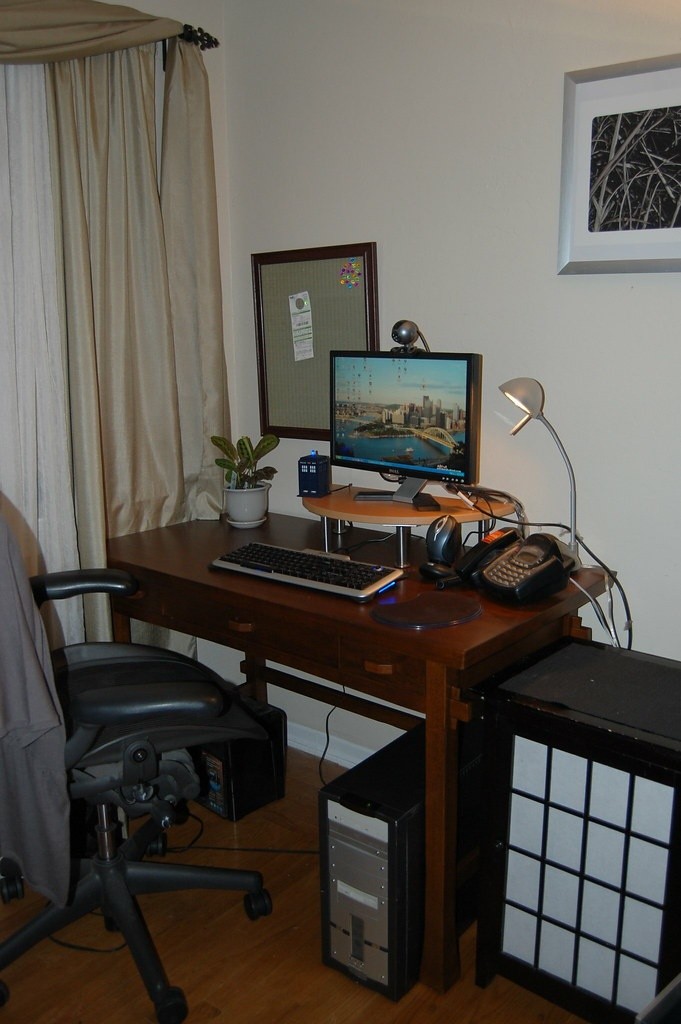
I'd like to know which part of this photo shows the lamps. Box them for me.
[497,377,582,572]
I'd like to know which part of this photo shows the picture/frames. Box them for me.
[250,242,380,442]
[556,54,681,276]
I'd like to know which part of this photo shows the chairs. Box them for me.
[0,518,274,1024]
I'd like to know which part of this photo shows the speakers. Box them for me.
[425,515,462,568]
[197,697,287,823]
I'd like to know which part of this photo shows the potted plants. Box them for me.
[211,433,280,529]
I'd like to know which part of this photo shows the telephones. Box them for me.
[454,526,575,604]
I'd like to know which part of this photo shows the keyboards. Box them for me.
[208,541,404,602]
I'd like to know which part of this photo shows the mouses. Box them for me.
[419,562,457,581]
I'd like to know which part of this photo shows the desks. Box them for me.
[105,513,617,997]
[301,485,515,569]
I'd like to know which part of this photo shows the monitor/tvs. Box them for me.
[330,350,483,511]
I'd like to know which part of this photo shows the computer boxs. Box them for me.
[318,722,488,1003]
